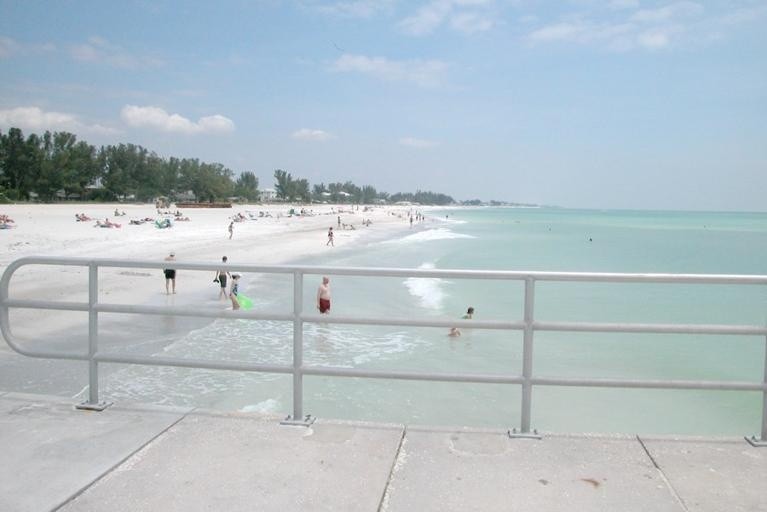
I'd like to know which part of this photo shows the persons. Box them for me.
[316,276,331,315]
[0,214,14,228]
[450,328,461,336]
[461,306,474,320]
[229,272,243,310]
[163,252,175,294]
[214,256,232,301]
